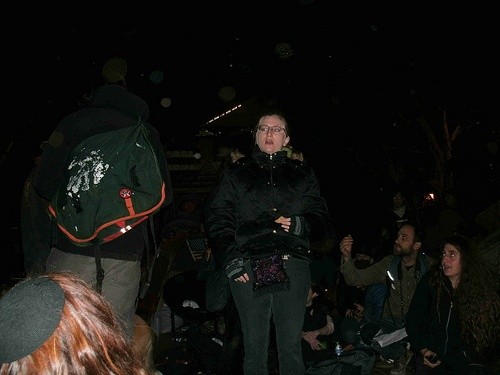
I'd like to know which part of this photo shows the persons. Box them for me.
[405,233,500,375]
[162,190,420,361]
[0,271,164,375]
[30,55,174,315]
[207,112,331,375]
[338,223,438,375]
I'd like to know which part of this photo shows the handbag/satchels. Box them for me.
[46,112,173,247]
[360,319,408,359]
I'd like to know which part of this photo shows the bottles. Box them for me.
[334,341,342,360]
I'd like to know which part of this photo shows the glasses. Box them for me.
[259,125,285,133]
